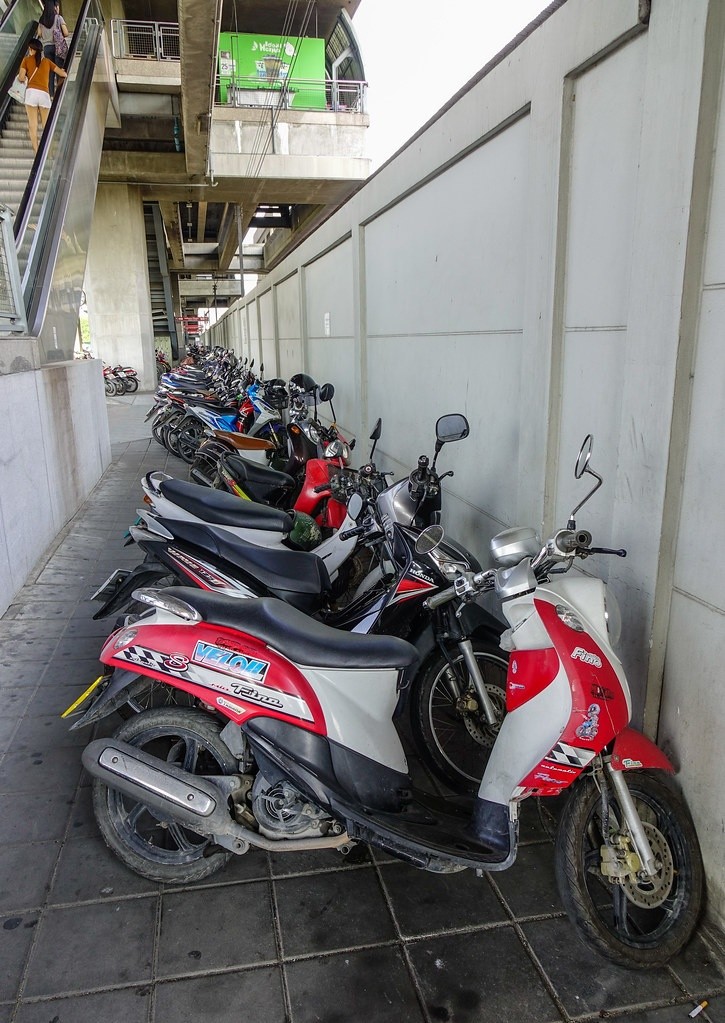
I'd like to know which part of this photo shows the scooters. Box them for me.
[62,430,706,974]
[89,342,512,800]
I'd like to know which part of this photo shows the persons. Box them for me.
[38,0,69,100]
[19,39,68,152]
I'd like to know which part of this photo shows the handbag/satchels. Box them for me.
[7,73,28,103]
[52,29,68,59]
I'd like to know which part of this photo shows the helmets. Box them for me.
[264,378,288,399]
[285,508,322,552]
[289,373,317,396]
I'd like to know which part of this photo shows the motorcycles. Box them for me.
[76,352,140,398]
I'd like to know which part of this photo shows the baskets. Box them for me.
[264,395,288,410]
